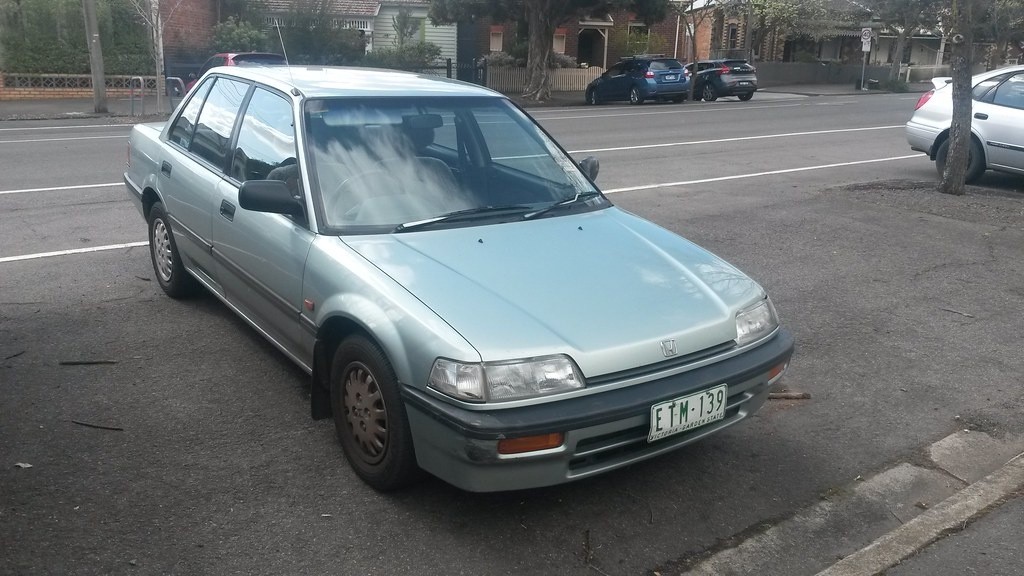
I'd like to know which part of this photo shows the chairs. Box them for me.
[373,157,460,195]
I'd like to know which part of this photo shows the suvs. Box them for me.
[585,56,692,106]
[185,51,289,93]
[683,58,759,102]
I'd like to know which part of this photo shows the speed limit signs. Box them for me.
[861,27,873,41]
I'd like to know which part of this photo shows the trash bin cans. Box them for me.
[817,57,841,84]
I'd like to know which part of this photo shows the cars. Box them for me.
[903,64,1024,184]
[121,64,798,494]
